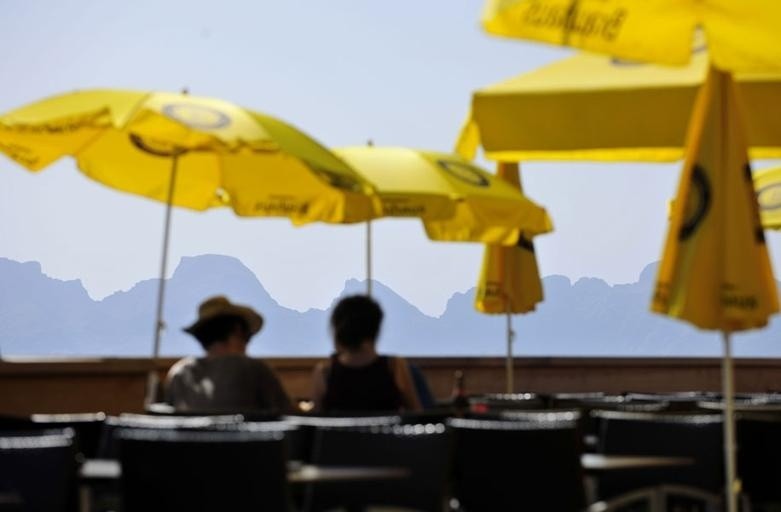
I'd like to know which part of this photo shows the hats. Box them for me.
[184,296,262,335]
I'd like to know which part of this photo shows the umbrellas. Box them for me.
[475,155,547,395]
[450,51,781,172]
[0,84,392,406]
[650,62,781,512]
[472,0,781,69]
[328,134,556,296]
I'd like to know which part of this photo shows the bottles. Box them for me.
[450,370,470,419]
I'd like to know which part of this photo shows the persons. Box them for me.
[309,292,425,415]
[164,294,294,422]
